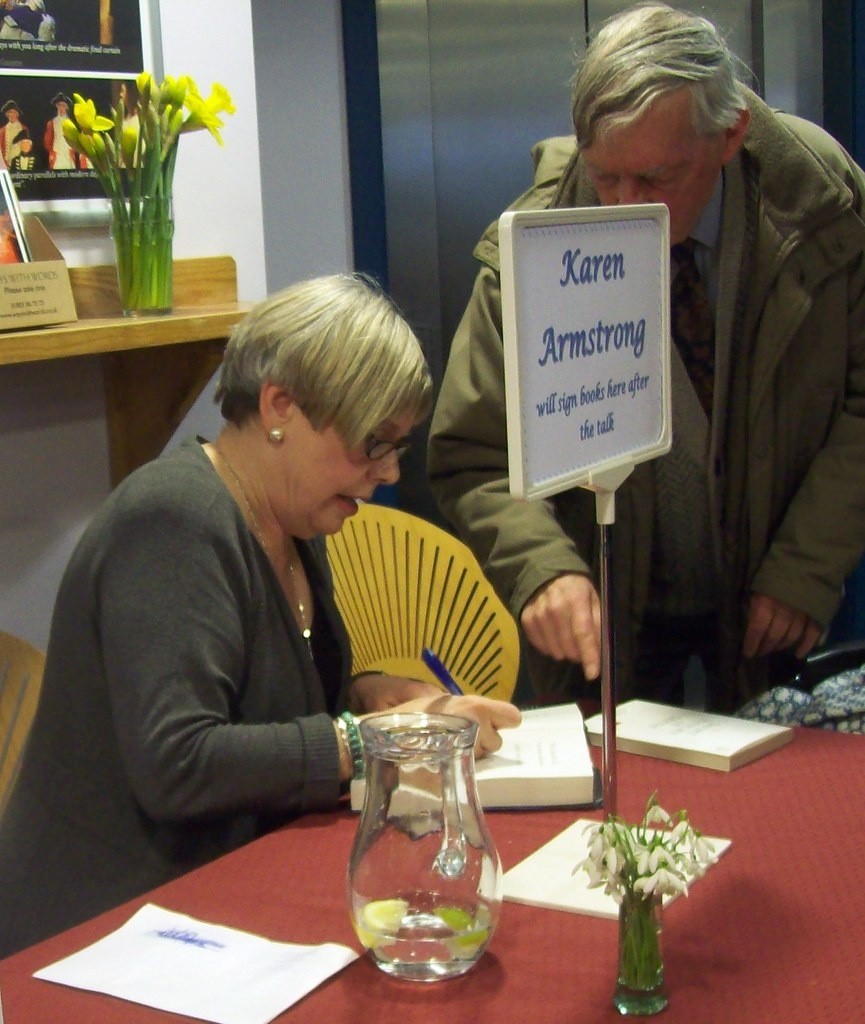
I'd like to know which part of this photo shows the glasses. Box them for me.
[362,432,413,462]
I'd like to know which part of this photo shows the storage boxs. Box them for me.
[0,216,80,331]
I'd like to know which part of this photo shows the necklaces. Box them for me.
[233,471,313,663]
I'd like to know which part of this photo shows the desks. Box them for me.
[0,699,865,1024]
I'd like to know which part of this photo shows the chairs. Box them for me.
[0,631,51,812]
[326,504,523,704]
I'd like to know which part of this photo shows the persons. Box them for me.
[1,274,521,960]
[422,5,865,717]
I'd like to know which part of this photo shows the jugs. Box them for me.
[345,712,504,982]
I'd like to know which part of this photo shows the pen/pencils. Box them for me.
[421,646,465,697]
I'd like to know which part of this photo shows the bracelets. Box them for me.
[336,712,364,777]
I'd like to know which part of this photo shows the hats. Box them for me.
[1,101,24,121]
[51,93,72,107]
[13,130,36,144]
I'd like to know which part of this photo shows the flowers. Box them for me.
[60,74,237,312]
[577,792,720,986]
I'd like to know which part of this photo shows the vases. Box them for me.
[104,194,175,318]
[613,893,670,1015]
[343,709,506,985]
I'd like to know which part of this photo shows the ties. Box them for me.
[670,237,716,426]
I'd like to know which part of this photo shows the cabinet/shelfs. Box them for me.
[0,256,256,487]
